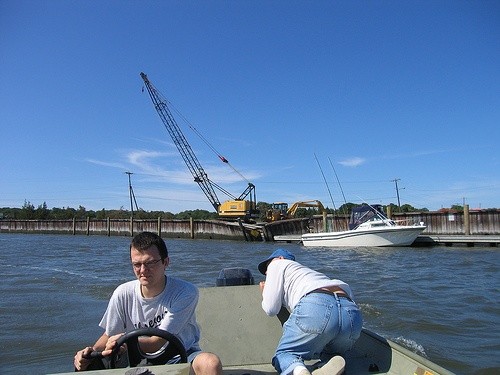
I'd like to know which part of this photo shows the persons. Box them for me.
[257,249,362,375]
[75,232,222,375]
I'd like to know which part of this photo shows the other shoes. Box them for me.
[293,365,312,375]
[311,355,345,375]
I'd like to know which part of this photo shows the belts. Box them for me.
[306,289,353,302]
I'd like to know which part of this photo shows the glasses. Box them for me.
[133,259,163,270]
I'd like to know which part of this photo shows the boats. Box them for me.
[301,152,427,247]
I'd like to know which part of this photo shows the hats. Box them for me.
[257,248,295,276]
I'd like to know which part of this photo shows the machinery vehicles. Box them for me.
[263,200,325,221]
[140,71,261,222]
